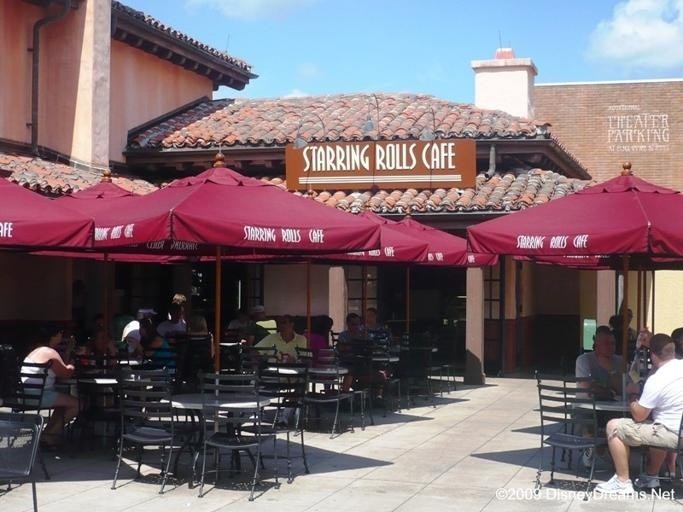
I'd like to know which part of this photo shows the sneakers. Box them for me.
[374,398,385,407]
[279,407,293,426]
[633,473,661,488]
[595,473,635,494]
[581,448,593,468]
[663,472,675,483]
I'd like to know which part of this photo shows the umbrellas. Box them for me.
[515,256,682,332]
[465,160,682,369]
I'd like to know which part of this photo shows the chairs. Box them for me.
[310,325,456,440]
[531,368,610,501]
[561,376,597,472]
[635,348,653,371]
[636,411,683,497]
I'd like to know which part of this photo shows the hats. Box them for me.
[252,305,265,313]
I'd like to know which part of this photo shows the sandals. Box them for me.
[39,428,66,451]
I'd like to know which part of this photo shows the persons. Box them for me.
[572,309,682,468]
[18,302,191,459]
[593,333,682,494]
[229,306,430,409]
